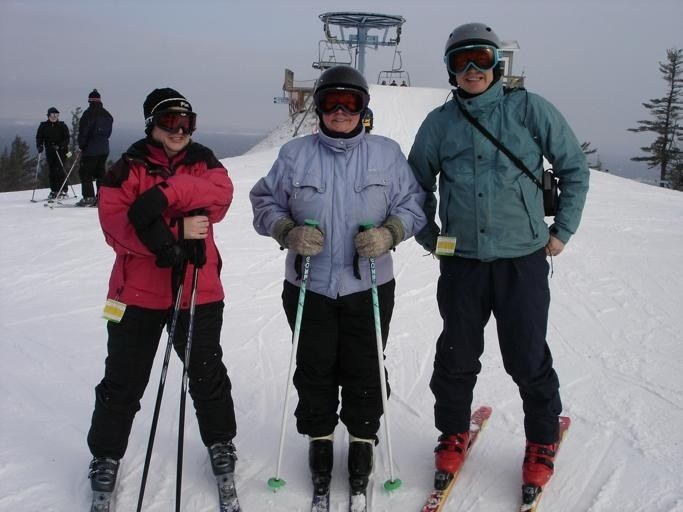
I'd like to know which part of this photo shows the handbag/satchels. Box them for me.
[542,169,560,216]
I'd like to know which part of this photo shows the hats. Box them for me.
[88,88,101,102]
[143,87,192,125]
[47,107,59,116]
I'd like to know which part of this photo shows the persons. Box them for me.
[85,85,238,494]
[380,79,386,85]
[405,21,592,504]
[248,62,427,496]
[35,106,70,198]
[77,88,113,205]
[399,79,406,86]
[389,79,397,85]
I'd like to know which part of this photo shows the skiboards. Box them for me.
[310,474,368,512]
[90,473,240,512]
[30,196,97,208]
[420,406,569,512]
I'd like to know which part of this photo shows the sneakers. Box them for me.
[89,454,127,491]
[48,191,68,199]
[305,430,337,476]
[346,431,380,476]
[75,196,99,207]
[206,438,239,479]
[520,434,558,488]
[432,427,471,475]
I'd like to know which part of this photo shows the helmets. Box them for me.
[313,62,371,117]
[442,21,504,57]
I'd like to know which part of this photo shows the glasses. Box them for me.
[155,110,196,134]
[442,44,500,75]
[49,112,59,117]
[314,87,369,116]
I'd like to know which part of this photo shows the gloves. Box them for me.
[36,144,45,152]
[272,217,326,257]
[56,141,66,151]
[127,184,169,229]
[352,215,406,260]
